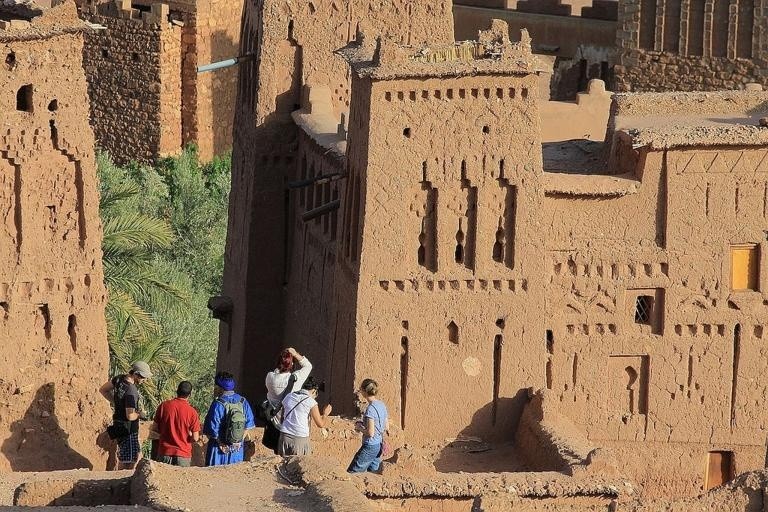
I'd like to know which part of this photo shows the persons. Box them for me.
[97,361,153,470]
[276,375,332,455]
[261,346,314,443]
[346,378,392,473]
[202,369,257,466]
[153,380,200,466]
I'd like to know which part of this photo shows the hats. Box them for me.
[133,361,153,378]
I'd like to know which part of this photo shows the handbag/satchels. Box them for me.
[107,420,130,440]
[260,400,275,423]
[379,441,390,458]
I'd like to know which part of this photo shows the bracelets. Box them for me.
[294,352,299,356]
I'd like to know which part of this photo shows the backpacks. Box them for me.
[213,396,246,446]
